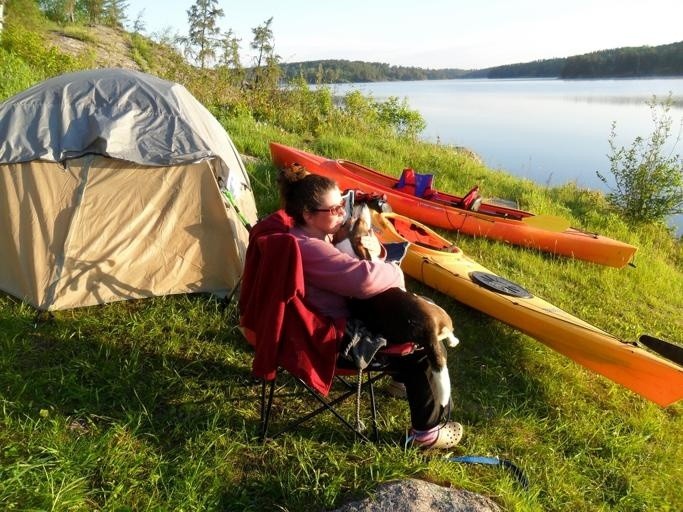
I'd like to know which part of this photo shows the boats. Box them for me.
[346,187,683,411]
[269,137,637,273]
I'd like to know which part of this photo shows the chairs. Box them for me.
[234,209,418,449]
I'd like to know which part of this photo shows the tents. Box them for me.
[0,67,259,313]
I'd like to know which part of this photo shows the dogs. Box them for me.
[330,203,459,425]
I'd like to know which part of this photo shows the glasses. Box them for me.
[313,200,346,215]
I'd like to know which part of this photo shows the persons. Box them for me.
[283,175,463,452]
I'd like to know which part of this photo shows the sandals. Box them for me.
[410,422,463,449]
